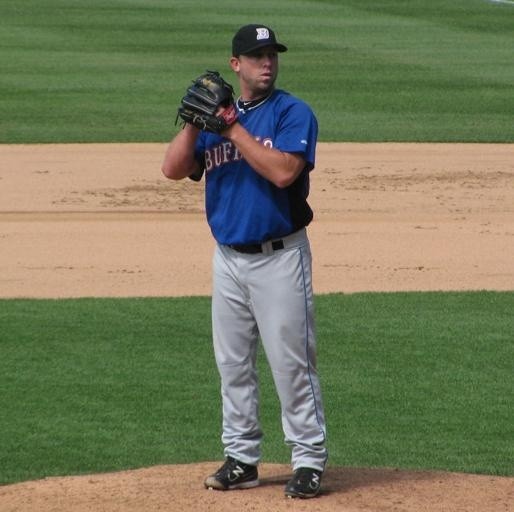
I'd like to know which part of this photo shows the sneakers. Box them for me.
[283,467,322,500]
[204,456,260,490]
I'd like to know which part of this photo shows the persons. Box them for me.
[159,23,330,501]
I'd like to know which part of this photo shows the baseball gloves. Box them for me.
[177,70,241,133]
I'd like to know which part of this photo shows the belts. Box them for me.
[215,239,283,254]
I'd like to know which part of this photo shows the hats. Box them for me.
[232,23,288,56]
